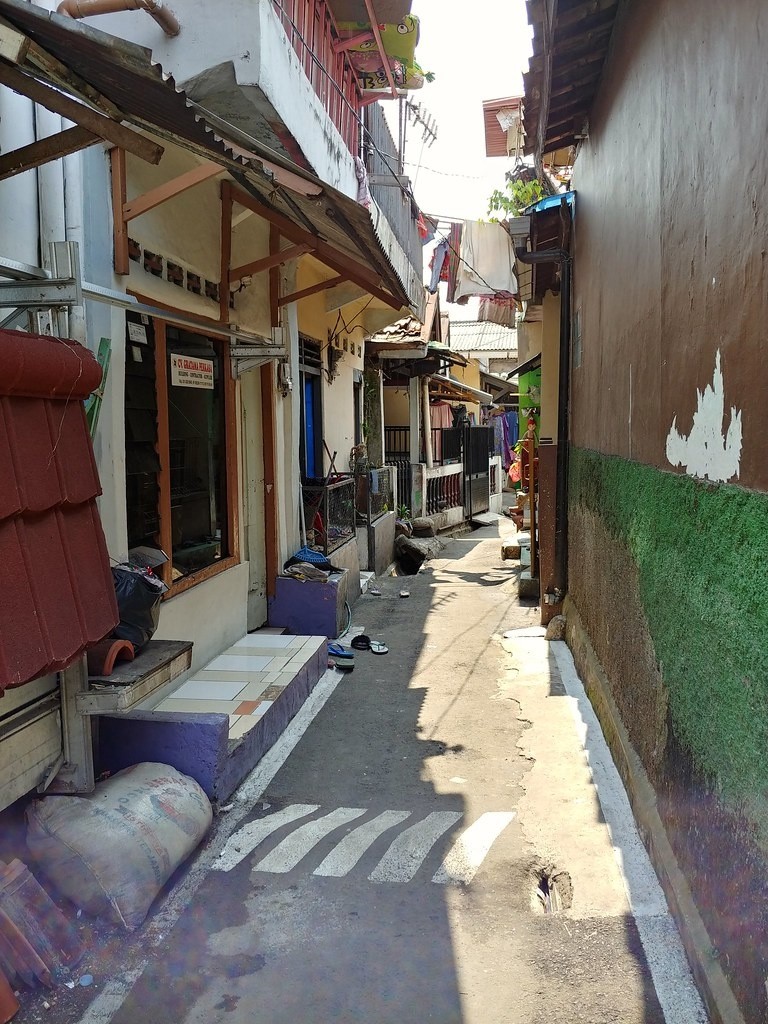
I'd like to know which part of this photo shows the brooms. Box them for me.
[282,481,346,575]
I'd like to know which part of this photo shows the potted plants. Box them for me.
[489,181,545,250]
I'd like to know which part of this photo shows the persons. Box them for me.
[522,420,538,444]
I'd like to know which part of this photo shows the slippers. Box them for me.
[328,636,389,669]
